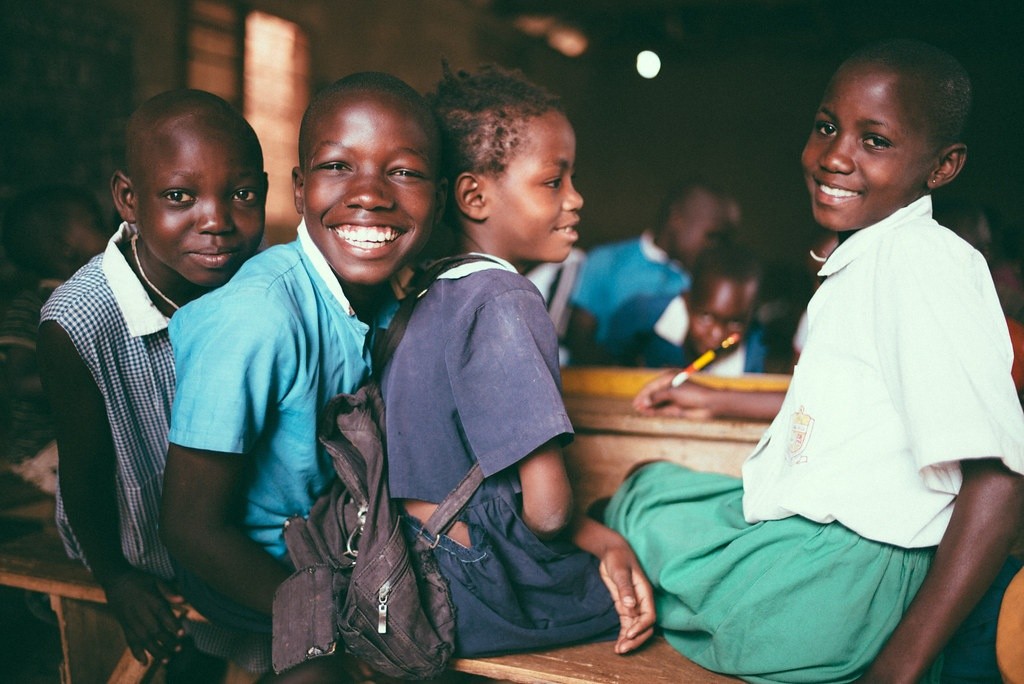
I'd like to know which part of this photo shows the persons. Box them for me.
[158,71,441,684]
[0,87,268,684]
[378,65,657,654]
[558,41,1024,684]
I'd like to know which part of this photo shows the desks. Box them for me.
[556,389,778,510]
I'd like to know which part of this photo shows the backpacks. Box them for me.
[270,253,508,682]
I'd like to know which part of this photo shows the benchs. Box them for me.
[0,526,750,684]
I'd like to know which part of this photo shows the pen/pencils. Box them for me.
[670,332,741,388]
